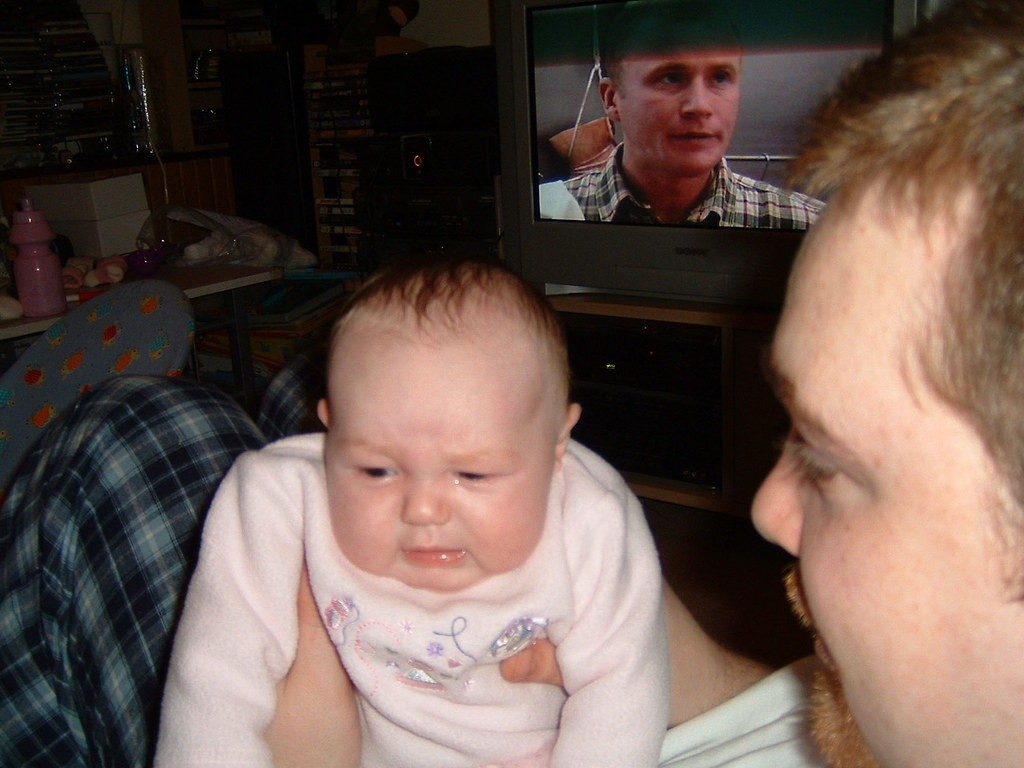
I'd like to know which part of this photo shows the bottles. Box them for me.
[10,196,67,317]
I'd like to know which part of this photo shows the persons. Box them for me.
[560,0,825,230]
[0,0,1024,768]
[153,251,669,768]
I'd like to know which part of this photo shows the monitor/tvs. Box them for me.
[492,0,919,309]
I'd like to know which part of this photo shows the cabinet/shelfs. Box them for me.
[546,291,791,519]
[140,0,232,150]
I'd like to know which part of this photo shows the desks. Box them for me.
[0,250,282,408]
